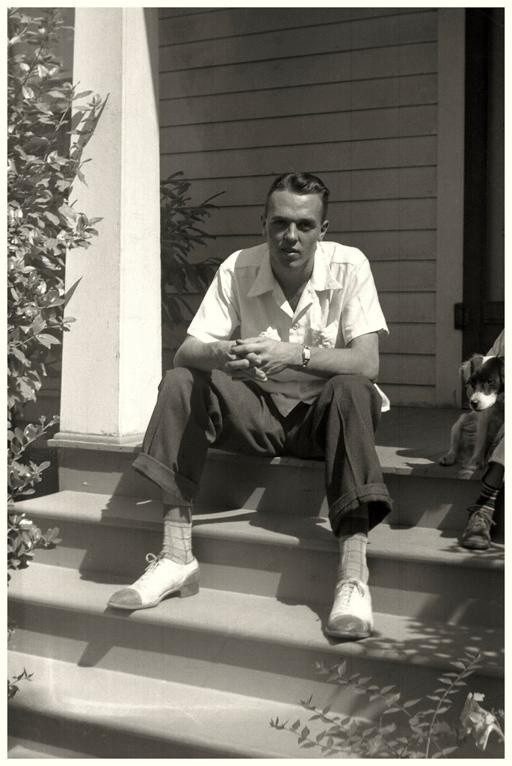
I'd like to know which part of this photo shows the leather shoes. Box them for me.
[327,577,373,638]
[107,554,201,611]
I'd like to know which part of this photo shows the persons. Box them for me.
[459,326,505,549]
[107,173,392,644]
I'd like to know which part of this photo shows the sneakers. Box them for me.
[460,504,495,549]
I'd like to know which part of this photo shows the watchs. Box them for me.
[297,344,312,369]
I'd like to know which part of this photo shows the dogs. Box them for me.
[436,353,504,471]
